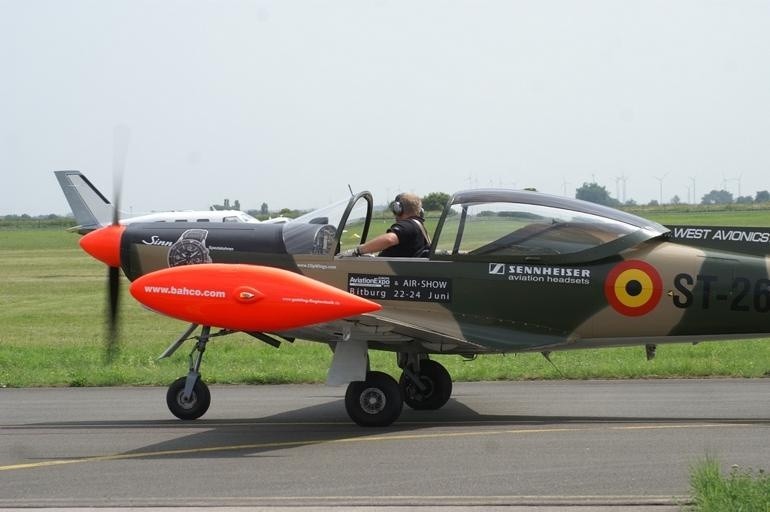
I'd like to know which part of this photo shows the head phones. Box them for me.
[389,193,424,218]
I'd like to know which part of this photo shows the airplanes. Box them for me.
[55,169,291,232]
[78,126,770,428]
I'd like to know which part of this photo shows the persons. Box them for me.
[337,191,432,256]
